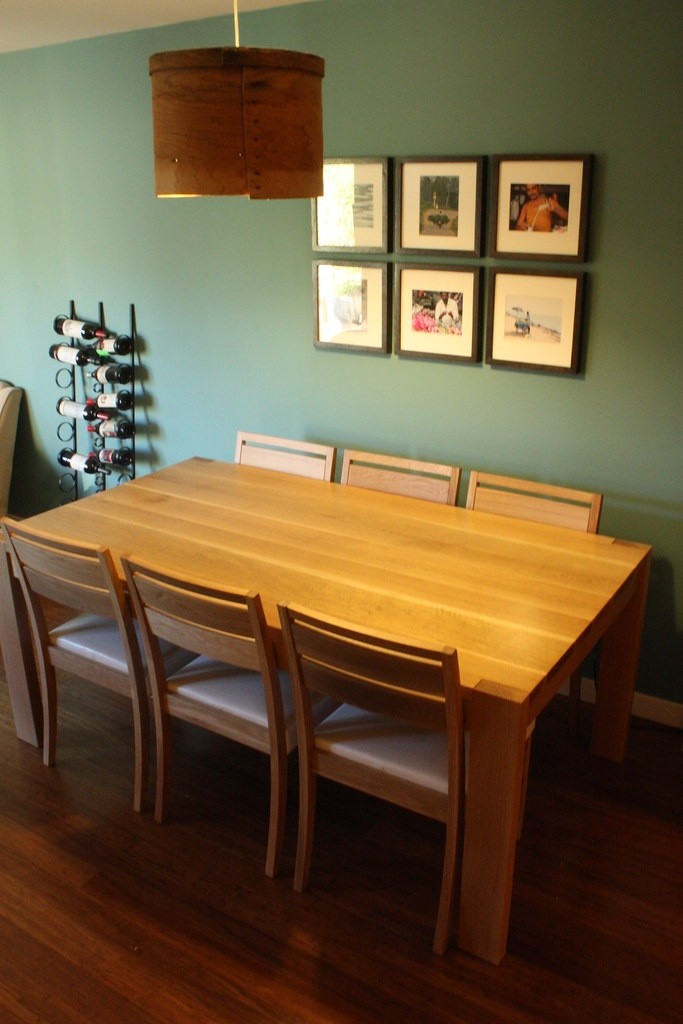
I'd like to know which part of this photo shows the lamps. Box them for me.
[149,0,325,201]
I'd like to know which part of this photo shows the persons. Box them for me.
[516,184,568,232]
[526,311,530,333]
[412,290,463,327]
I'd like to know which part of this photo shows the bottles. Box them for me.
[86,393,133,411]
[57,450,112,476]
[55,398,111,421]
[87,339,131,356]
[86,365,132,384]
[48,344,103,367]
[87,450,131,466]
[53,318,110,340]
[87,421,134,440]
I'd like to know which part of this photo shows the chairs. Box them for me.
[1,515,202,814]
[275,599,537,957]
[120,550,342,878]
[235,432,604,739]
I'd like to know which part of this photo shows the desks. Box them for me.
[0,455,652,966]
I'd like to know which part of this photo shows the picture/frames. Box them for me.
[486,266,585,373]
[489,153,592,264]
[394,155,489,258]
[393,261,486,363]
[310,156,393,255]
[311,257,392,356]
